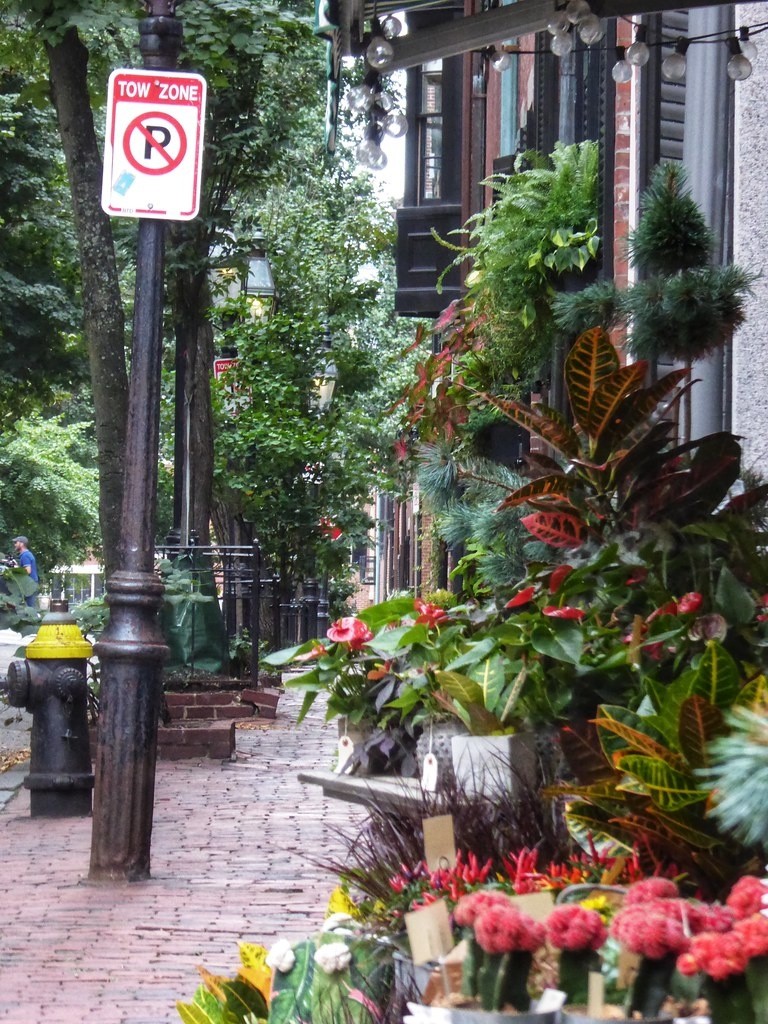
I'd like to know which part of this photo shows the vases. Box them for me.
[337,716,374,749]
[416,720,467,786]
[451,731,537,801]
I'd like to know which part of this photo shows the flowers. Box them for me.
[175,565,768,1024]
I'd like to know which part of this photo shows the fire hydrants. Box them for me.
[0,598,96,794]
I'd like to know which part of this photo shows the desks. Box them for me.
[297,771,434,820]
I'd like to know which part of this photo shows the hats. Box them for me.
[12,536,28,546]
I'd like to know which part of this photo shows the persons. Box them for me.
[12,536,38,608]
